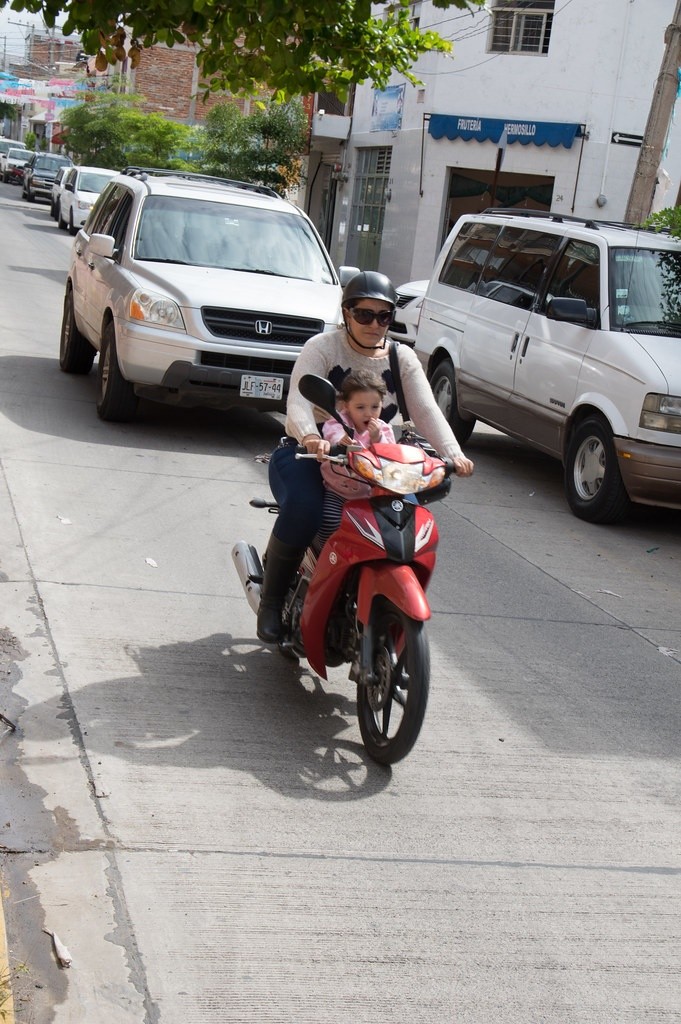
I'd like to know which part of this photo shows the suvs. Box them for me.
[412,207,681,524]
[58,165,343,422]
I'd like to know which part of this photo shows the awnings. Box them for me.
[427,112,584,149]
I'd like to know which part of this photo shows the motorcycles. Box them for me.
[231,373,473,765]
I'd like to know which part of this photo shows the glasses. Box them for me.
[348,307,396,328]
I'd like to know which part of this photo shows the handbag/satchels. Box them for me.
[395,419,452,506]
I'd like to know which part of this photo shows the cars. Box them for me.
[21,151,76,202]
[49,167,73,218]
[58,165,121,235]
[2,147,34,183]
[0,135,27,181]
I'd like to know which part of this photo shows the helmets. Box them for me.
[341,271,397,312]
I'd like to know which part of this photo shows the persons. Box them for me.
[318,370,396,539]
[256,272,474,644]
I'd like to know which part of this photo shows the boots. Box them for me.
[257,532,305,644]
